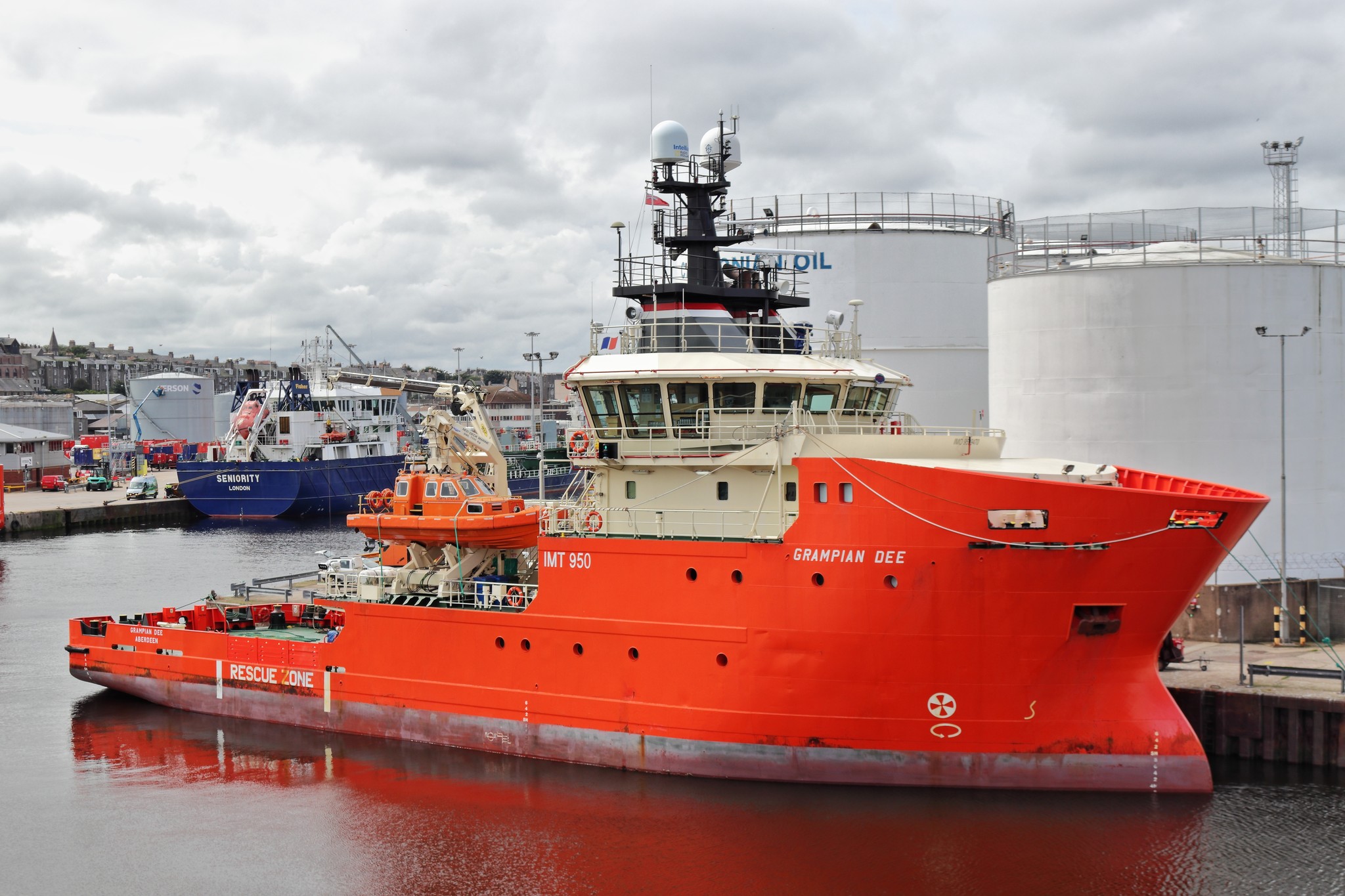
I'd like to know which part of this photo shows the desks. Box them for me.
[606,416,622,435]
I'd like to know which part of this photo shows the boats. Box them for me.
[319,430,347,441]
[346,452,569,550]
[64,63,1272,794]
[235,401,270,440]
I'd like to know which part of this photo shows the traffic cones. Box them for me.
[163,493,167,499]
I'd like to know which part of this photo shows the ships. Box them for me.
[176,325,588,518]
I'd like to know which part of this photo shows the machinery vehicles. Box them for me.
[86,462,114,491]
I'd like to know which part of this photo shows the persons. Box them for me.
[75,470,81,483]
[164,483,173,498]
[406,441,413,450]
[157,386,160,389]
[161,386,164,395]
[304,456,309,462]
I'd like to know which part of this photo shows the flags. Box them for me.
[645,193,669,206]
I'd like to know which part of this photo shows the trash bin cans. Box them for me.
[164,483,185,497]
[501,575,520,598]
[1172,639,1184,655]
[486,575,510,606]
[520,446,527,450]
[473,577,496,606]
[891,421,901,434]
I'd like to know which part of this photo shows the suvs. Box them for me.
[41,475,68,492]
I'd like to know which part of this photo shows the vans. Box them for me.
[126,476,158,501]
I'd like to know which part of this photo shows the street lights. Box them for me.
[101,458,104,468]
[524,331,540,438]
[453,348,465,385]
[1255,325,1312,643]
[522,352,559,500]
[347,344,357,377]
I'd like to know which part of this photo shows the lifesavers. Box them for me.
[570,430,588,452]
[586,512,602,532]
[304,457,308,461]
[381,488,394,509]
[366,490,383,509]
[507,586,523,606]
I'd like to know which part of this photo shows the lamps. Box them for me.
[632,469,771,475]
[1096,465,1107,474]
[1062,464,1074,474]
[720,195,727,210]
[763,208,774,220]
[826,310,844,333]
[602,444,609,459]
[1003,212,1013,218]
[652,169,659,182]
[658,188,664,193]
[625,306,644,324]
[355,527,359,533]
[693,177,698,183]
[599,493,603,496]
[1081,235,1088,241]
[725,140,732,154]
[593,322,603,332]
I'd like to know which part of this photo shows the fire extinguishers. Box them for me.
[655,279,657,284]
[633,421,639,427]
[654,170,657,182]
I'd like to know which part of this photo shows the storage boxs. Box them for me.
[63,435,221,465]
[280,440,288,444]
